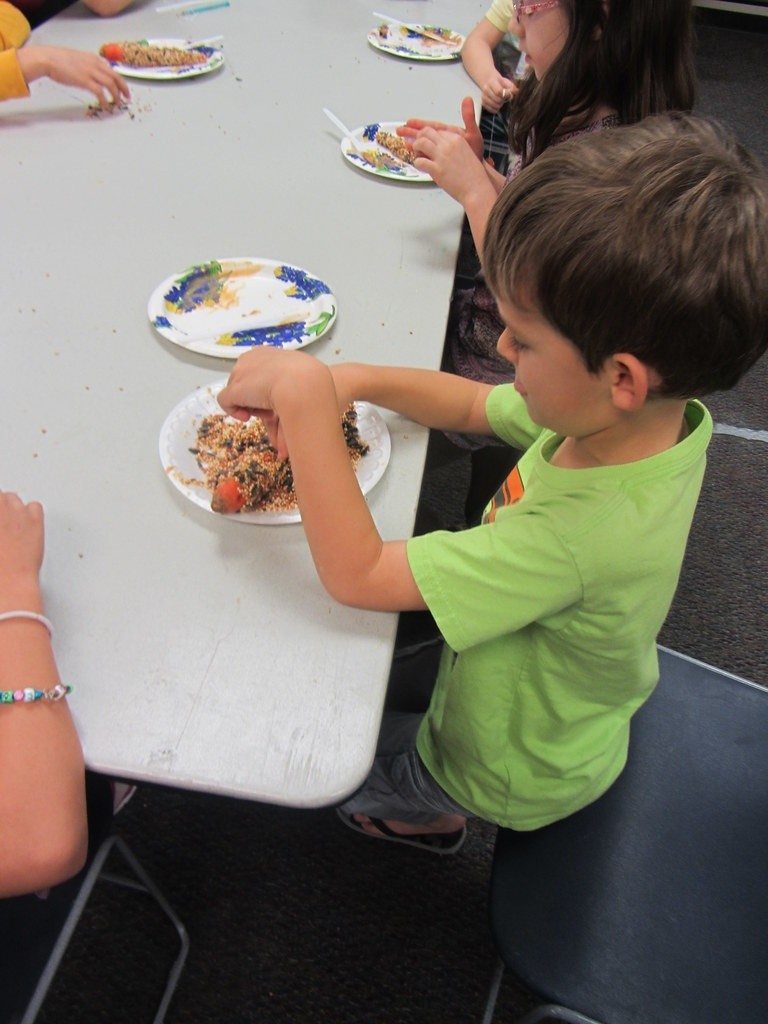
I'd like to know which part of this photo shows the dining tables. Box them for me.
[0,0,491,816]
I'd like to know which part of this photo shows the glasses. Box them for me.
[513,0,564,24]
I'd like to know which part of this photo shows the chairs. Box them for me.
[476,642,768,1024]
[1,831,194,1024]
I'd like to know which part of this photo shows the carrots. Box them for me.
[210,478,246,515]
[99,43,124,60]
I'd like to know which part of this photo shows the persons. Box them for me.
[396,0,693,529]
[0,490,137,898]
[218,109,768,853]
[0,0,130,112]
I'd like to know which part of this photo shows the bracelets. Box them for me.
[0,685,71,703]
[0,610,54,639]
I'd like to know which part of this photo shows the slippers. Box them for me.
[336,805,467,855]
